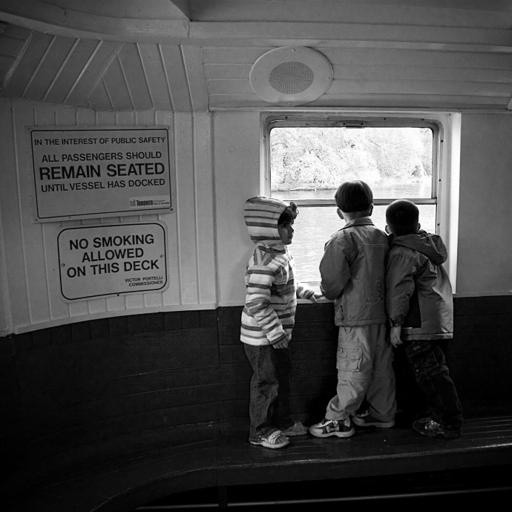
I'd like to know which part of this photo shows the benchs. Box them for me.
[0,294,512,511]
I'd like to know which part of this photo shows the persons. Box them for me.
[241,196,322,450]
[382,200,465,440]
[306,180,397,438]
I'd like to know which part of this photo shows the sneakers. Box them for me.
[309,406,460,439]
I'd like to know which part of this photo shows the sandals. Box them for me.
[249,420,308,449]
